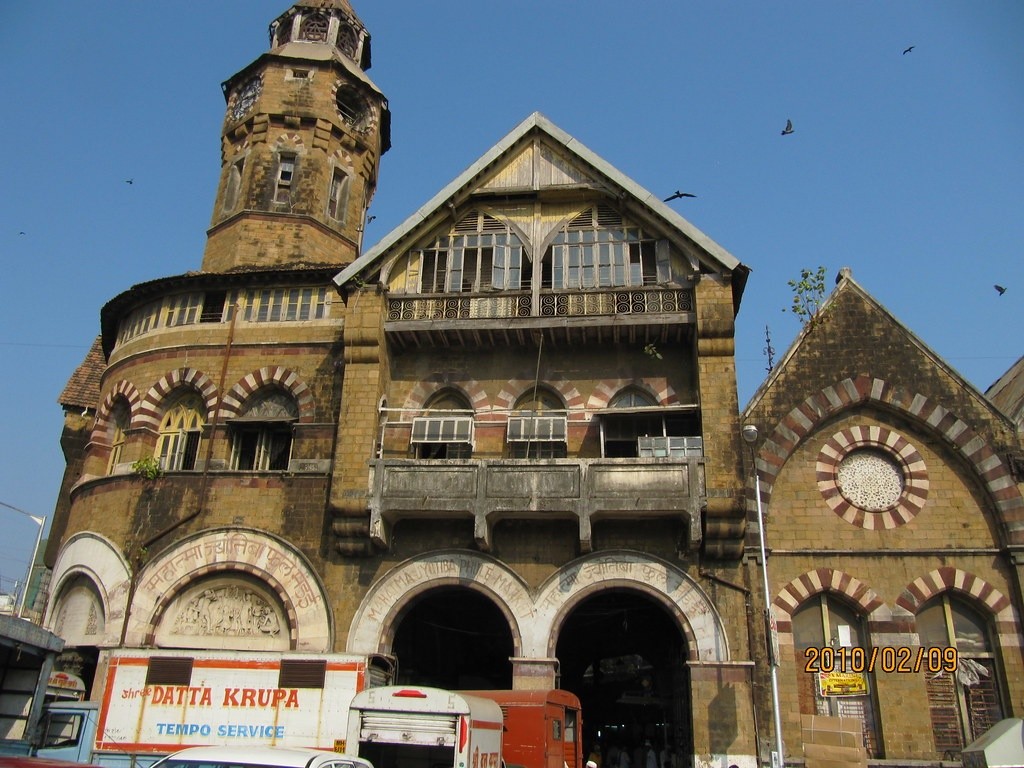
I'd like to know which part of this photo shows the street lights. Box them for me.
[741,424,787,768]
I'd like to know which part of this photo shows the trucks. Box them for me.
[344,685,508,768]
[31,646,398,768]
[449,688,582,768]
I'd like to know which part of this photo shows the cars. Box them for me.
[149,743,374,768]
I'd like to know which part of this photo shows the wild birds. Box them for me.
[126,178,134,185]
[19,231,25,236]
[902,45,915,56]
[781,118,795,137]
[366,214,377,224]
[664,190,696,203]
[992,283,1008,297]
